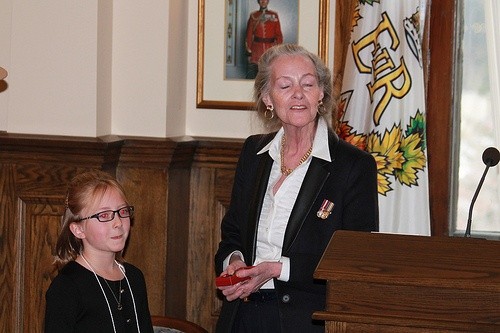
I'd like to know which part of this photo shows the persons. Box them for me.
[43,170,155,333]
[214,42,379,333]
[244,0,284,78]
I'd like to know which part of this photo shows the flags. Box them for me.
[331,1,429,238]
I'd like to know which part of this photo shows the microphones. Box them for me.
[464,147,500,237]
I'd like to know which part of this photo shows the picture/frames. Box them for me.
[196,0,326,110]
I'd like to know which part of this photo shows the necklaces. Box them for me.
[279,127,312,176]
[82,252,123,310]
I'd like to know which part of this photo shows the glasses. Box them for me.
[73,205,135,222]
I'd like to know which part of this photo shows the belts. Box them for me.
[241,291,278,303]
